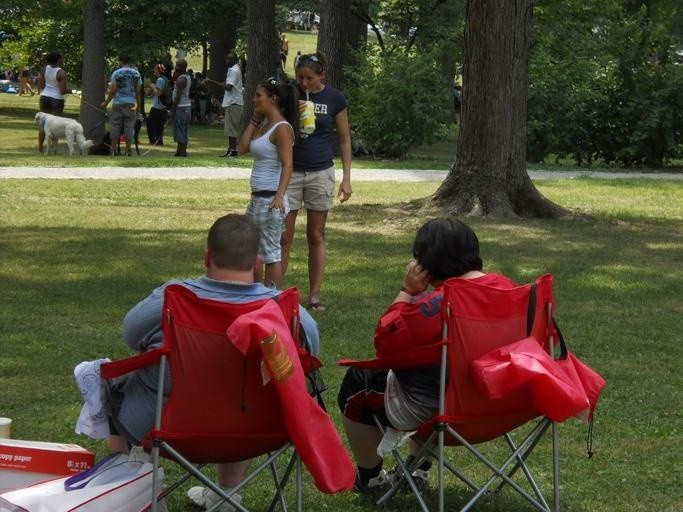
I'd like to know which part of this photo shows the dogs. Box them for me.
[34,112,95,156]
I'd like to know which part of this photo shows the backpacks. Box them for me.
[159,76,173,107]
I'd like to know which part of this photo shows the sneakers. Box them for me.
[392,463,430,492]
[186,485,243,512]
[345,468,391,494]
[220,150,238,158]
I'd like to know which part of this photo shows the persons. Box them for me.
[294,50,301,69]
[237,76,299,290]
[106,213,321,511]
[0,53,243,158]
[280,52,352,311]
[282,33,289,70]
[337,217,519,495]
[286,12,319,35]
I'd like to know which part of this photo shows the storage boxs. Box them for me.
[0,438,94,495]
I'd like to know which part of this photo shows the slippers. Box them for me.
[308,297,326,313]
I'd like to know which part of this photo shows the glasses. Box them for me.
[299,55,324,71]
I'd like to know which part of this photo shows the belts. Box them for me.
[251,190,277,198]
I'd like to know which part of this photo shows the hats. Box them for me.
[156,63,167,75]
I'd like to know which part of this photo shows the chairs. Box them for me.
[336,269,567,507]
[104,280,324,511]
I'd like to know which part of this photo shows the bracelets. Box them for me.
[399,287,417,297]
[251,118,258,126]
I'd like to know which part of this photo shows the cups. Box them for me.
[299,101,315,135]
[0,416,10,438]
